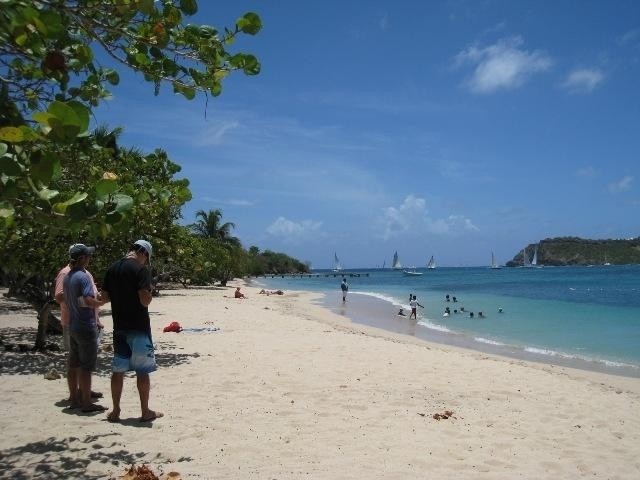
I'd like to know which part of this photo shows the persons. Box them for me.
[235,287,246,298]
[259,288,283,295]
[443,307,482,318]
[409,294,424,319]
[55,240,163,422]
[498,308,503,312]
[341,279,349,301]
[446,294,458,302]
[398,308,407,316]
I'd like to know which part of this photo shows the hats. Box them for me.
[133,239,153,267]
[69,243,95,256]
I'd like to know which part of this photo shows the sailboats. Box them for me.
[331,252,345,273]
[518,243,544,269]
[489,247,503,269]
[390,249,436,276]
